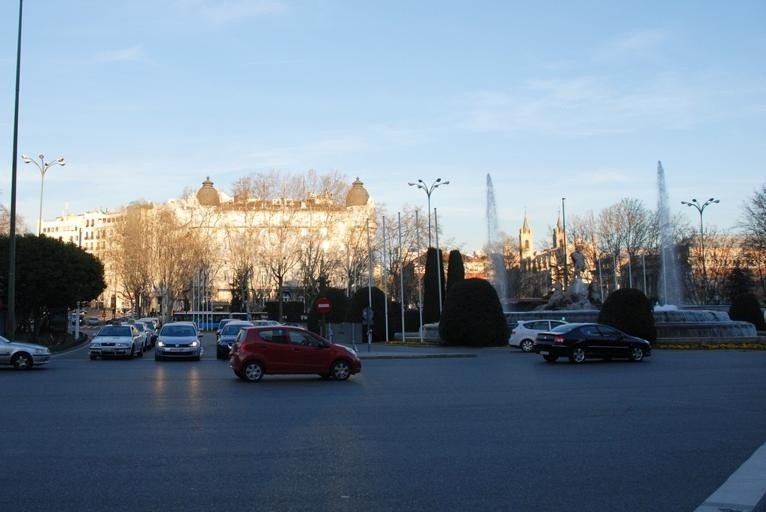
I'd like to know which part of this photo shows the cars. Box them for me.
[508,319,651,364]
[0,335,52,371]
[88,318,361,382]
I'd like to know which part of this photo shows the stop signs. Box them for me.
[315,297,332,312]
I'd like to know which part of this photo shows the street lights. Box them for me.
[21,153,65,237]
[408,178,449,248]
[681,198,720,274]
[561,192,567,292]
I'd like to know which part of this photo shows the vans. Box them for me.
[72,313,81,325]
